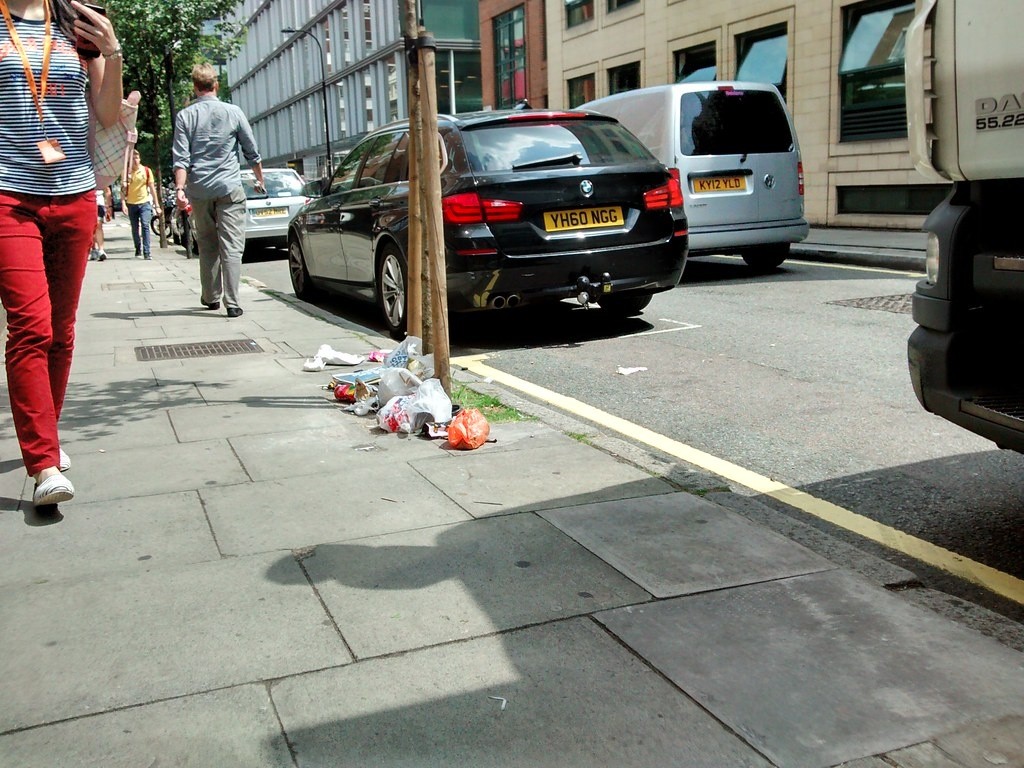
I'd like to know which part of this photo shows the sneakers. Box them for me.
[135,251,141,258]
[60,449,70,472]
[32,474,74,506]
[200,296,220,310]
[144,254,152,259]
[97,251,107,261]
[227,308,243,316]
[90,249,97,260]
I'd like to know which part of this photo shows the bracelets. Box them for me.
[107,205,113,208]
[153,206,160,208]
[176,185,186,191]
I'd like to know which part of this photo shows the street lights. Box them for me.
[281,26,332,177]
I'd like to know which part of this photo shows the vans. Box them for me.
[582,81,809,271]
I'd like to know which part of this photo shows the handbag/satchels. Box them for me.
[85,90,141,189]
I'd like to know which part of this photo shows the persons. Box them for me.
[88,189,113,257]
[174,63,266,315]
[120,149,163,260]
[0,1,125,503]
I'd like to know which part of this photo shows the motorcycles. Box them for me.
[151,201,176,238]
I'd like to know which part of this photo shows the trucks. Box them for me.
[907,0,1024,454]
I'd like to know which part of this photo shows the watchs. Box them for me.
[102,45,123,59]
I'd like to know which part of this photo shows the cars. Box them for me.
[285,107,690,342]
[240,168,318,249]
